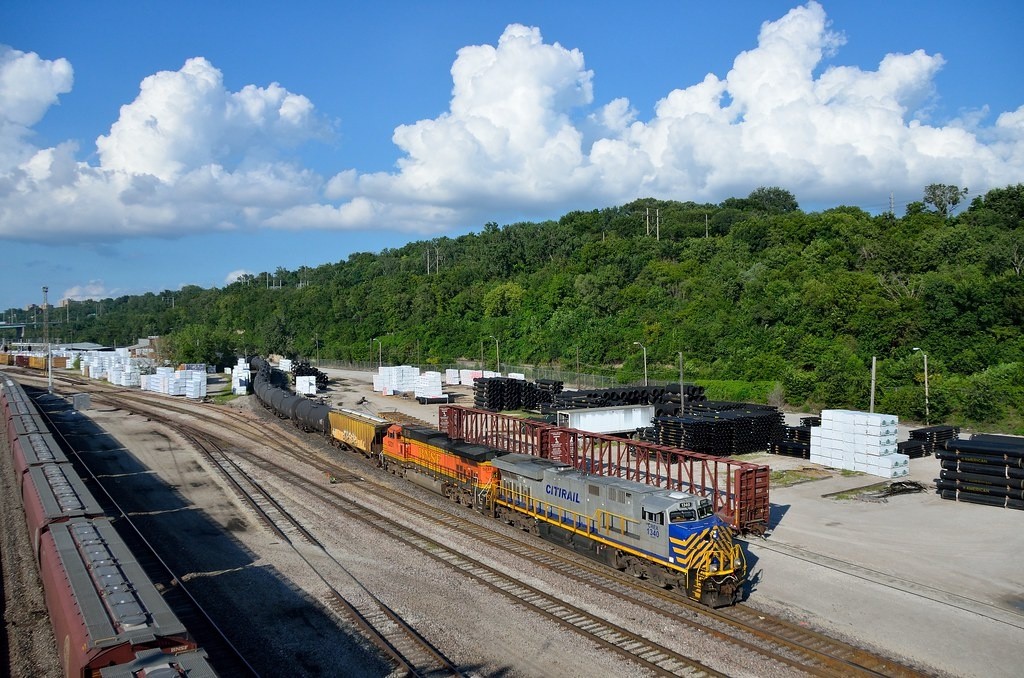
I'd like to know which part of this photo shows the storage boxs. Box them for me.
[296,376,317,395]
[278,359,292,372]
[372,365,525,398]
[0,350,251,399]
[809,409,910,478]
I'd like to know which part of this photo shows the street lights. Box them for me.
[373,338,381,367]
[490,336,499,373]
[633,342,647,387]
[311,338,319,367]
[912,347,929,428]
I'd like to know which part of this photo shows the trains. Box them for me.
[252,356,750,611]
[0,371,219,678]
